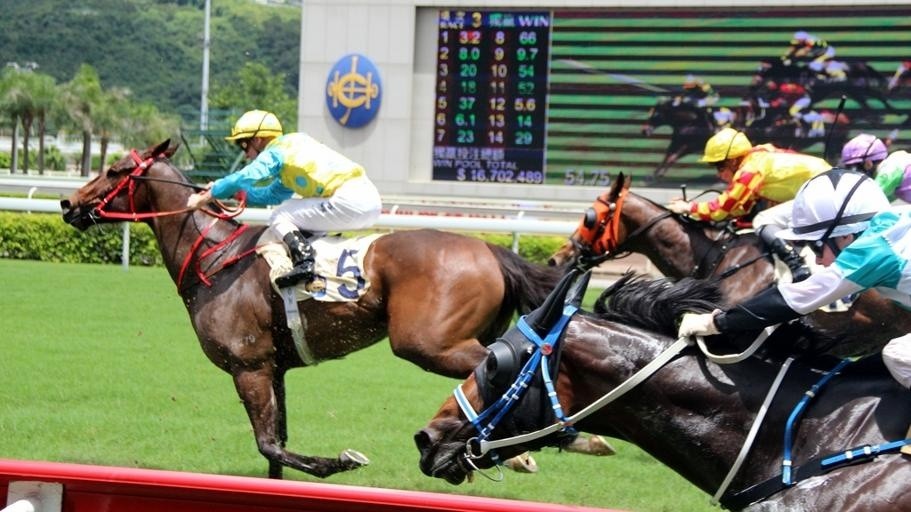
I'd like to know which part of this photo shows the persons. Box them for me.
[749,55,780,98]
[669,126,832,223]
[840,130,910,205]
[758,82,816,120]
[677,74,724,110]
[677,170,910,397]
[183,110,385,287]
[782,31,849,84]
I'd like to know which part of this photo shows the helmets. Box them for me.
[223,109,285,143]
[838,130,890,167]
[699,126,753,165]
[775,167,892,243]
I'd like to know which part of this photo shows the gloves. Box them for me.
[676,307,725,341]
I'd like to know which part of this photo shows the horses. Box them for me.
[633,53,910,181]
[409,170,911,511]
[58,136,617,478]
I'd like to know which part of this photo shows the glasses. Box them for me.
[237,137,254,151]
[716,162,727,174]
[808,239,828,260]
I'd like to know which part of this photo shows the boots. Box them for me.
[757,224,812,283]
[272,231,317,288]
